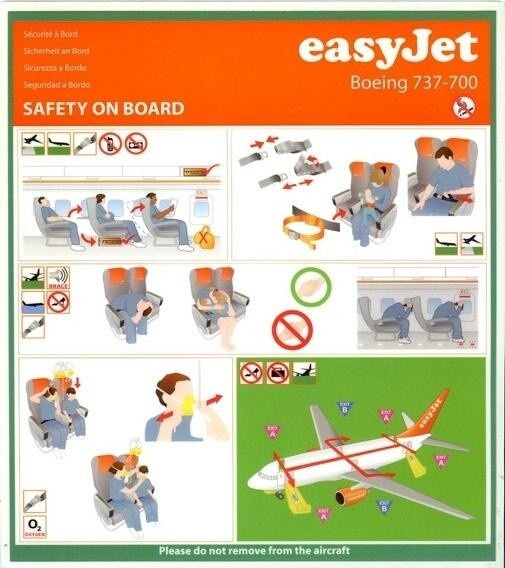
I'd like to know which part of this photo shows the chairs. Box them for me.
[91,455,145,531]
[407,137,478,217]
[358,297,401,340]
[412,296,455,340]
[32,197,180,246]
[104,266,162,337]
[332,163,400,245]
[26,377,88,452]
[189,267,251,340]
